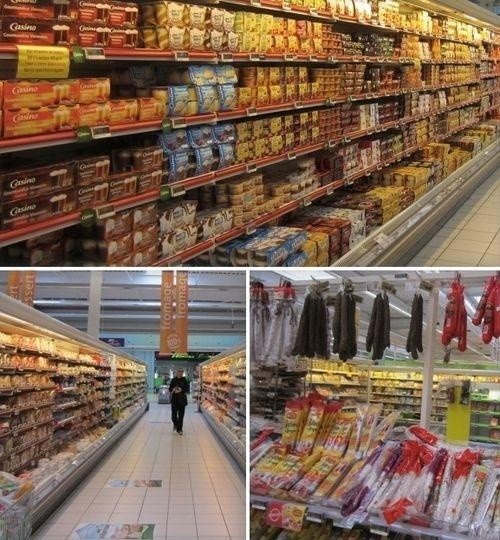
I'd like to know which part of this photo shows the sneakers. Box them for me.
[178,429,183,435]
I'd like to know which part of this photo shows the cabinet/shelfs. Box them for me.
[367,363,500,445]
[0,292,148,531]
[192,340,246,476]
[299,356,367,408]
[250,352,307,420]
[0,0,500,266]
[250,437,500,540]
[190,382,199,402]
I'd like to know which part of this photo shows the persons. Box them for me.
[169,368,190,435]
